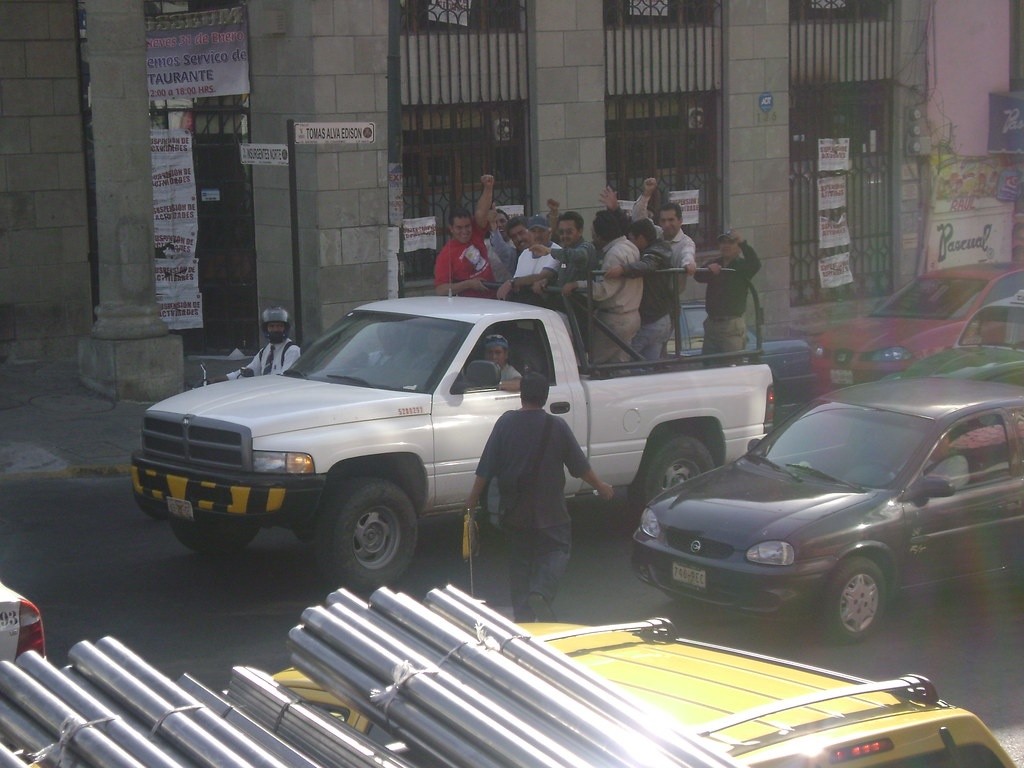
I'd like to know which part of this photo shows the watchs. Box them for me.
[498,380,502,391]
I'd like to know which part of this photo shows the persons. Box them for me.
[433,175,494,296]
[693,229,762,366]
[887,431,969,490]
[338,322,414,385]
[466,371,614,622]
[459,335,521,393]
[206,307,300,384]
[485,179,696,378]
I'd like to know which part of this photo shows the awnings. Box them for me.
[987,92,1024,155]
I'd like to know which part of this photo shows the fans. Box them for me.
[491,118,516,142]
[689,107,704,128]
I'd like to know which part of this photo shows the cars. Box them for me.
[0,577,45,661]
[632,376,1024,643]
[656,300,817,436]
[812,260,1024,394]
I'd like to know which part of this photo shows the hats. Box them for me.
[520,371,550,403]
[715,229,741,241]
[482,333,509,350]
[525,214,551,230]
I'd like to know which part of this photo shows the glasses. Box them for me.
[557,229,573,235]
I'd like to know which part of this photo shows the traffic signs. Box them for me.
[240,144,289,166]
[293,121,375,143]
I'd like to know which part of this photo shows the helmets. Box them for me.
[261,305,291,342]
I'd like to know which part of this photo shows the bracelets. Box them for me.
[509,278,515,288]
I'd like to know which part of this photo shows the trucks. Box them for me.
[273,623,1015,768]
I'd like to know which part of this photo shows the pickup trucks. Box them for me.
[131,295,773,594]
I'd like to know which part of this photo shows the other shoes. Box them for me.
[528,591,557,623]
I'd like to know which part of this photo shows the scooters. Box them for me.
[194,360,253,388]
